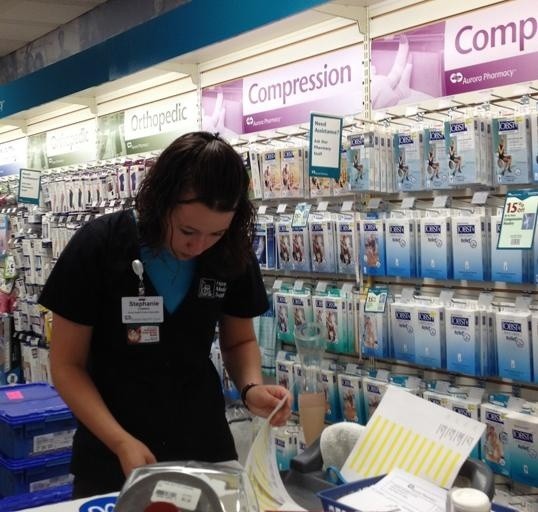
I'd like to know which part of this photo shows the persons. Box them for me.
[428,151,440,182]
[265,165,273,193]
[352,154,363,183]
[326,311,337,345]
[278,305,288,333]
[311,233,326,265]
[343,395,356,423]
[363,315,378,350]
[397,155,410,184]
[488,426,502,464]
[292,235,304,263]
[343,395,358,421]
[36,131,294,501]
[282,163,292,192]
[486,424,503,465]
[339,233,352,267]
[449,144,463,177]
[365,239,379,268]
[497,143,514,176]
[293,307,305,327]
[279,235,291,263]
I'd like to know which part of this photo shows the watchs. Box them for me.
[240,381,259,413]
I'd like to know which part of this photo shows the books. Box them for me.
[338,384,487,491]
[241,384,307,512]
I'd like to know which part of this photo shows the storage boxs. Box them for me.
[1,381,74,512]
